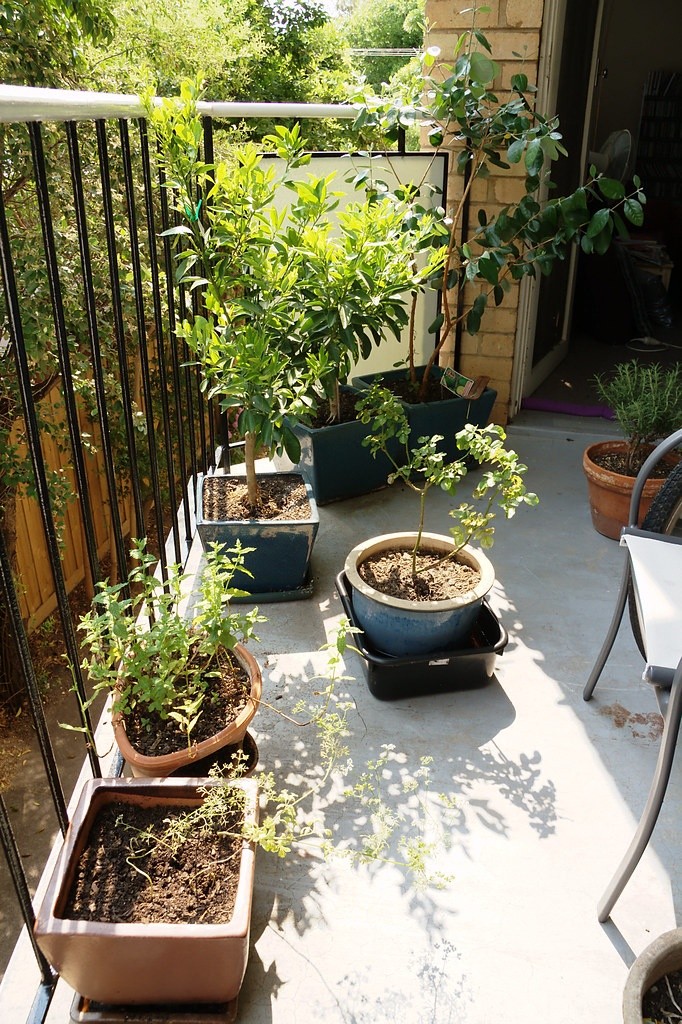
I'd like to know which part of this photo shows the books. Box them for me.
[616,236,669,266]
[630,69,682,200]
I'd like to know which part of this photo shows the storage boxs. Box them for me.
[336,569,511,703]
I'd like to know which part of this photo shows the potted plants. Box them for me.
[141,75,450,594]
[344,380,539,656]
[261,225,386,504]
[34,705,462,1006]
[69,536,266,777]
[583,359,682,542]
[622,927,682,1024]
[343,9,648,481]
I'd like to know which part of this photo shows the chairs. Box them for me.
[582,430,682,924]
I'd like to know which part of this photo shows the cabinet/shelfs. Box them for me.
[632,70,682,229]
